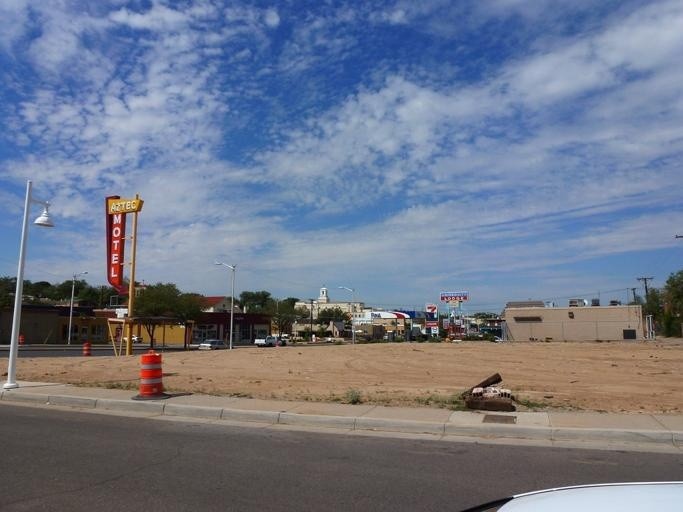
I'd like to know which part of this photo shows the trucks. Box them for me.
[253,336,276,346]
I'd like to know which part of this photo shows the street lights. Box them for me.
[2,179,54,391]
[338,286,355,344]
[215,258,236,350]
[66,271,88,344]
[308,298,315,341]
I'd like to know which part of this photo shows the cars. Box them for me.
[122,335,142,343]
[198,339,228,350]
